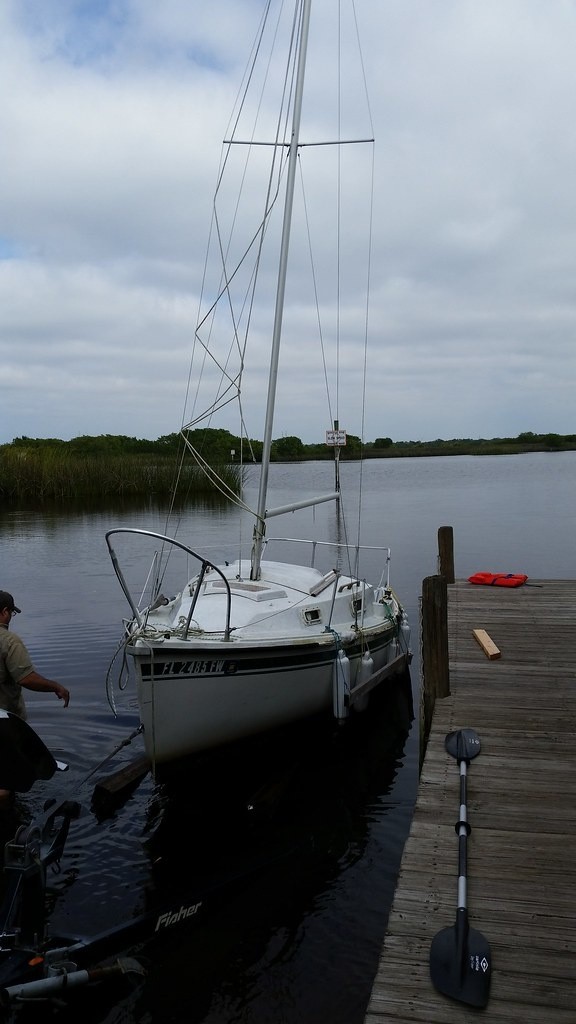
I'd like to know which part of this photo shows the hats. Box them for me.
[0,589,22,613]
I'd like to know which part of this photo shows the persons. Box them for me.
[0,590,70,722]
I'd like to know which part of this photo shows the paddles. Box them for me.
[429,726,490,1009]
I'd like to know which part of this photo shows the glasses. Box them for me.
[9,610,17,617]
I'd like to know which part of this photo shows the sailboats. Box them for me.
[104,0,413,770]
